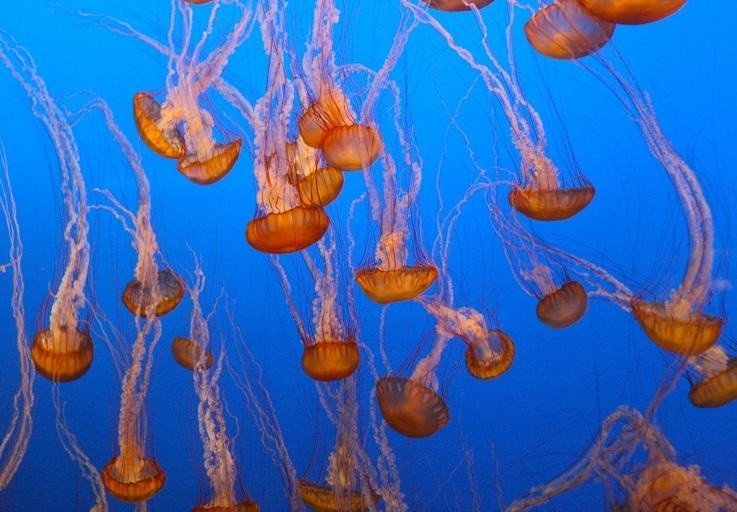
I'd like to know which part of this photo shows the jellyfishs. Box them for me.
[0,0,736,512]
